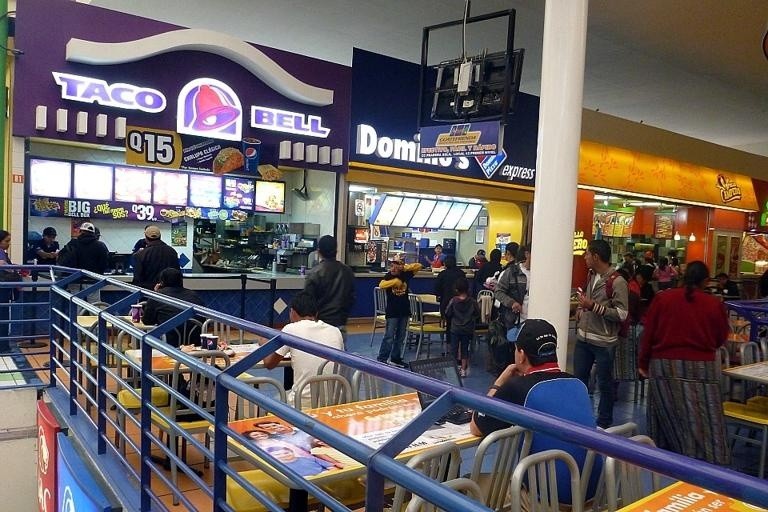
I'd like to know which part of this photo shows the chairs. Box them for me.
[75,272,768,512]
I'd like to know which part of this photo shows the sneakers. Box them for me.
[390,360,409,367]
[459,367,465,377]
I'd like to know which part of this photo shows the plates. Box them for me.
[206,209,248,221]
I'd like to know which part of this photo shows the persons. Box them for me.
[302,235,355,351]
[56,221,111,342]
[423,242,531,379]
[131,225,182,316]
[460,318,605,511]
[141,266,207,390]
[0,230,24,353]
[33,227,60,280]
[377,251,424,369]
[258,290,345,414]
[95,227,100,240]
[572,241,628,432]
[635,258,731,465]
[616,248,739,324]
[131,224,150,253]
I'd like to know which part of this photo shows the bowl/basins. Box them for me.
[276,263,287,272]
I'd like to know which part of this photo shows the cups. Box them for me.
[723,289,729,294]
[241,137,262,176]
[300,265,306,275]
[131,304,142,323]
[199,333,218,349]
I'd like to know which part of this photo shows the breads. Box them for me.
[212,147,245,176]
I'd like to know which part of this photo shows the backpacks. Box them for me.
[582,272,640,339]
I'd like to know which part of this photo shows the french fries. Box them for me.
[262,169,284,181]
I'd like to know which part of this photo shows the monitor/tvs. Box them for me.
[430,47,526,123]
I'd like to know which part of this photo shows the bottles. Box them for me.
[32,258,39,281]
[272,259,276,271]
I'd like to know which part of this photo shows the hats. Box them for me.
[387,251,407,265]
[43,222,160,239]
[507,319,557,357]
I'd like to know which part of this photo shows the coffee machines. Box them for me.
[345,225,371,274]
[264,222,320,272]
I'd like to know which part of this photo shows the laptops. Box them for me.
[409,358,473,425]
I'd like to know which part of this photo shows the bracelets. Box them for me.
[487,384,502,391]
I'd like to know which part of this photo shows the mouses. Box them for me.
[435,420,445,425]
